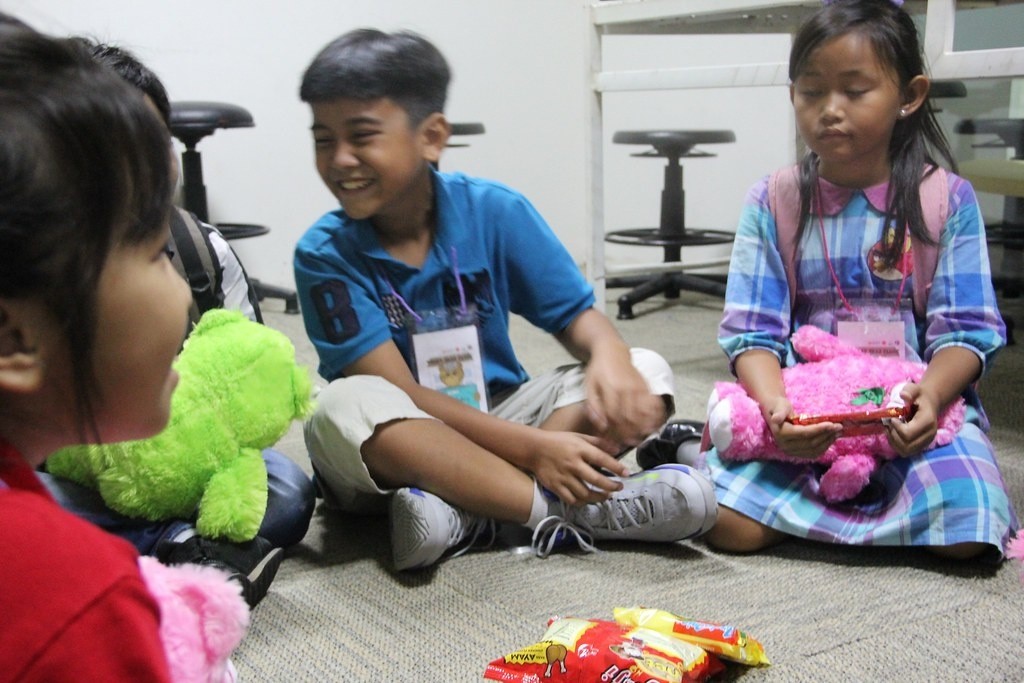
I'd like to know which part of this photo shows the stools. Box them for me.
[430,119,485,170]
[953,118,1024,299]
[927,82,967,114]
[169,100,299,314]
[603,129,736,322]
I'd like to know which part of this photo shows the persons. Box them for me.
[636,0,1014,563]
[0,28,191,683]
[291,26,722,569]
[32,39,315,609]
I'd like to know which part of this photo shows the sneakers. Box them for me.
[157,534,284,610]
[388,487,496,572]
[531,463,719,559]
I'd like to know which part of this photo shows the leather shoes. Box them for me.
[636,420,706,469]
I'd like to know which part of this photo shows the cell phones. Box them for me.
[790,406,912,438]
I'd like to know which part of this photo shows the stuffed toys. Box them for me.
[138,554,252,682]
[706,322,965,505]
[46,306,312,542]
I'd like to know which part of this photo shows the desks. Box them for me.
[581,0,1024,313]
